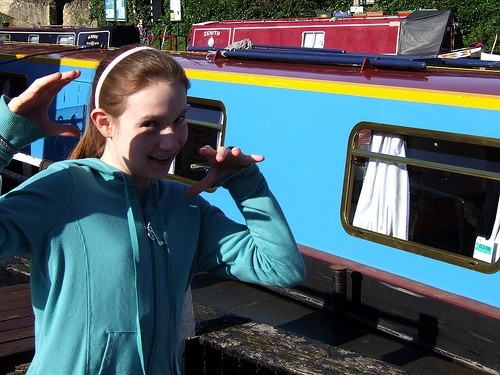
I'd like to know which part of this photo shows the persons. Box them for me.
[0,44,307,375]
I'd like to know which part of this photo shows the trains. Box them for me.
[191,10,429,60]
[1,40,500,374]
[0,24,141,47]
